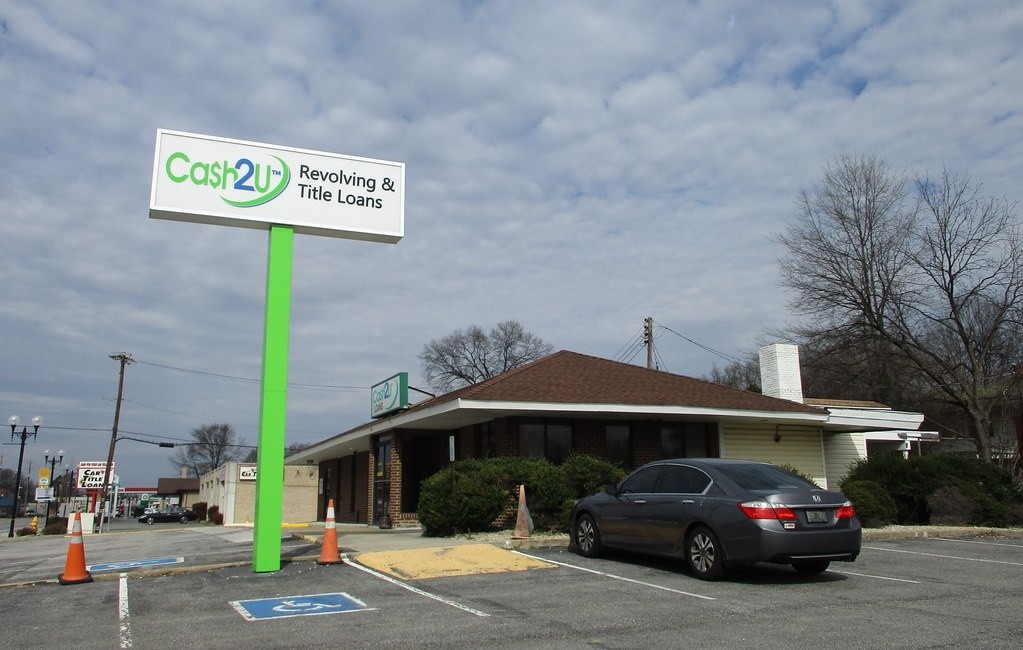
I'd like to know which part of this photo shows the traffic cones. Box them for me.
[316,499,344,565]
[58,511,93,586]
[511,485,534,539]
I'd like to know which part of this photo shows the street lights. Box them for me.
[45,448,64,525]
[7,414,43,537]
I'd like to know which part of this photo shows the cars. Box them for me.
[569,458,862,581]
[138,507,198,525]
